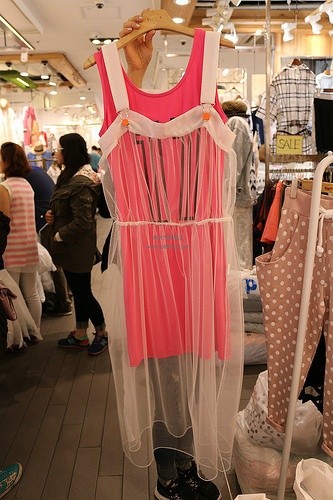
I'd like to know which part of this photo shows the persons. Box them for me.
[0,133,109,500]
[119,15,221,500]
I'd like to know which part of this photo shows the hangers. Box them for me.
[269,167,333,198]
[82,0,236,71]
[234,95,243,100]
[250,165,255,175]
[321,66,331,76]
[289,57,303,68]
[227,83,240,93]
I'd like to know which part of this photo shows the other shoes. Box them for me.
[22,335,36,345]
[46,303,72,316]
[5,342,29,352]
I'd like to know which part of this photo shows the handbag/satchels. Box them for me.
[0,284,18,321]
[244,368,323,455]
[232,411,309,500]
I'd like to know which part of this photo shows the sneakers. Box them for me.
[0,462,22,499]
[87,331,108,354]
[176,461,222,500]
[154,476,189,500]
[57,330,90,348]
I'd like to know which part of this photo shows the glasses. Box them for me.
[52,147,64,153]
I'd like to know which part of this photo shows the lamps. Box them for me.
[319,2,333,24]
[20,47,29,62]
[231,0,241,8]
[281,22,297,41]
[223,22,239,44]
[202,18,224,32]
[305,14,324,35]
[48,75,58,86]
[206,0,234,21]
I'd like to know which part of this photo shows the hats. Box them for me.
[220,102,250,118]
